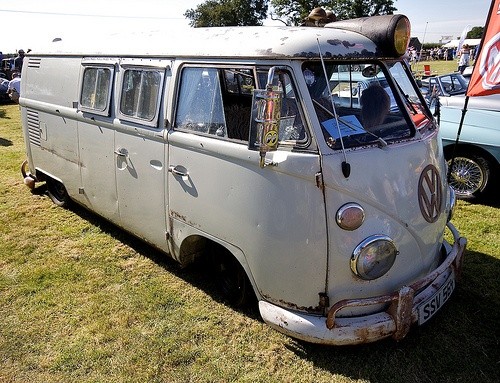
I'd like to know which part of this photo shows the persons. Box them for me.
[458,44,478,75]
[407,48,419,61]
[0,49,25,104]
[428,47,449,61]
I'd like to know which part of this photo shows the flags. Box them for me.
[466,0,500,97]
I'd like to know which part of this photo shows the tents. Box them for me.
[442,38,482,49]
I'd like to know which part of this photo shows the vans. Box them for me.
[19,14,467,346]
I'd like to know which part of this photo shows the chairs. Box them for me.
[224,93,341,142]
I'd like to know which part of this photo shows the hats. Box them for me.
[18,50,25,53]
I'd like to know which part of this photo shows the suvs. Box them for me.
[330,71,499,200]
